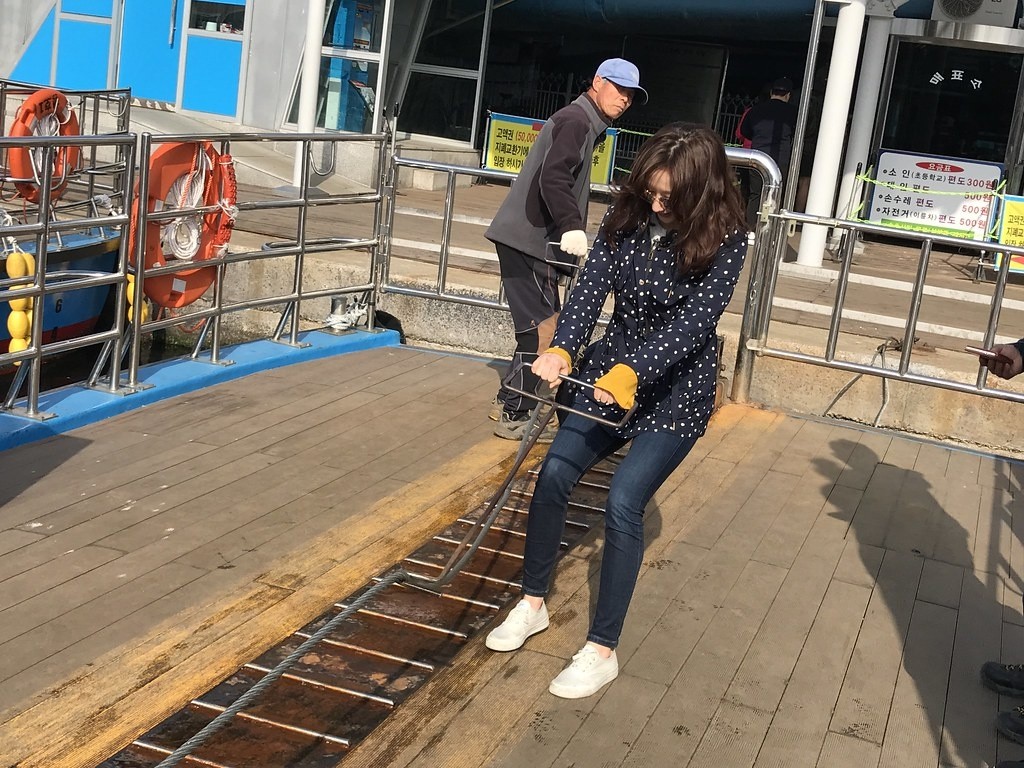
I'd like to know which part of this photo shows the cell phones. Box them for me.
[964,344,1014,366]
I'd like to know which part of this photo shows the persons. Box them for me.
[979,337,1024,744]
[484,59,648,443]
[735,77,794,244]
[485,125,747,700]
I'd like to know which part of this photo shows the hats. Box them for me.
[595,58,647,106]
[772,78,792,92]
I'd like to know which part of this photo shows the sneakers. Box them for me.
[995,706,1024,744]
[980,661,1024,699]
[548,646,619,699]
[996,759,1024,768]
[488,395,554,423]
[486,598,550,651]
[493,409,558,444]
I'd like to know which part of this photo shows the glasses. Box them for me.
[640,188,673,209]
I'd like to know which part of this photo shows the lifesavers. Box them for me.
[126,139,238,310]
[8,88,81,205]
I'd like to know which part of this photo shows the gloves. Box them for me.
[559,229,589,260]
